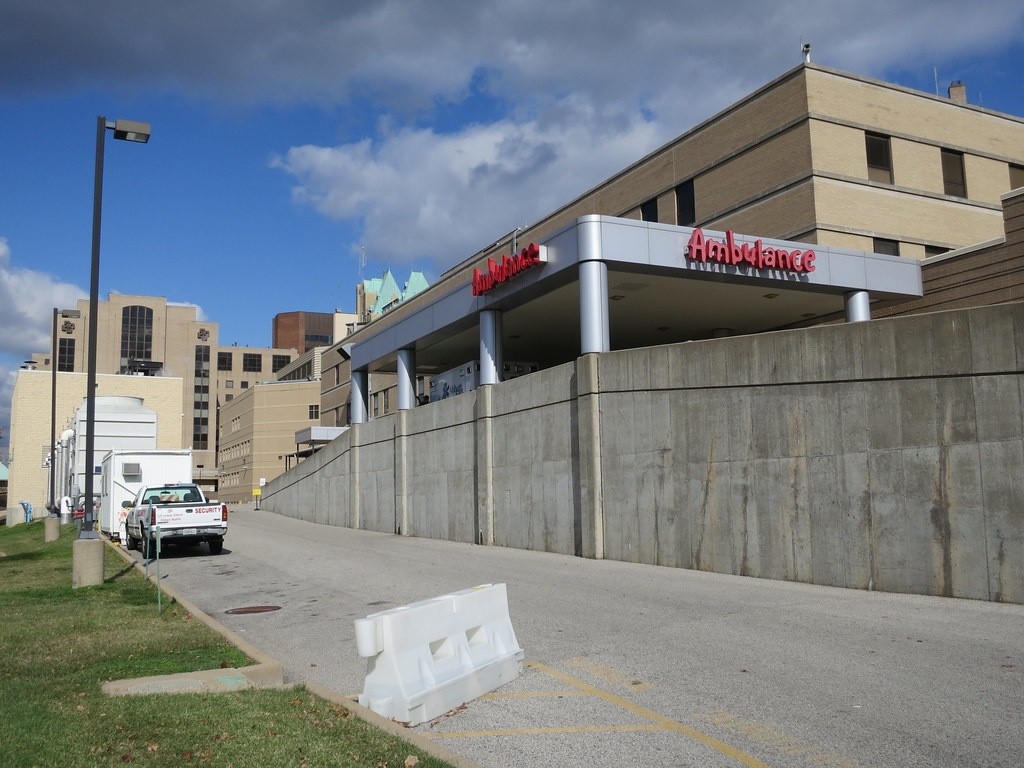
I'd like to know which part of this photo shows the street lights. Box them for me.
[76,111,153,539]
[47,306,81,518]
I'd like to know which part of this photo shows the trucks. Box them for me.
[98,448,195,543]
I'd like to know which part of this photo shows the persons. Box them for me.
[419,395,431,407]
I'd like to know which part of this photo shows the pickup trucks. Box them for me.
[121,482,228,559]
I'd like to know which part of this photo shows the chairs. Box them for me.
[149,496,159,504]
[184,494,195,502]
